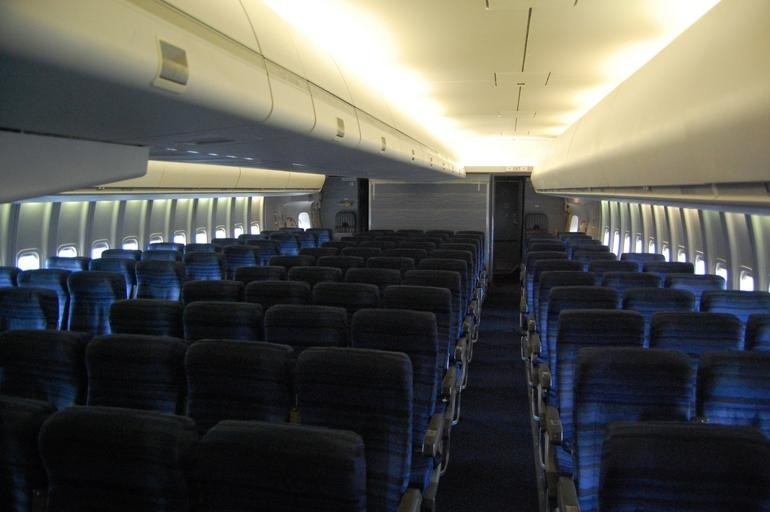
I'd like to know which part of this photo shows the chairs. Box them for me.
[199,419,366,508]
[560,346,698,509]
[2,400,47,511]
[38,405,195,509]
[698,352,767,430]
[352,308,447,508]
[601,420,767,511]
[536,309,647,476]
[520,225,768,419]
[0,229,483,423]
[294,344,422,508]
